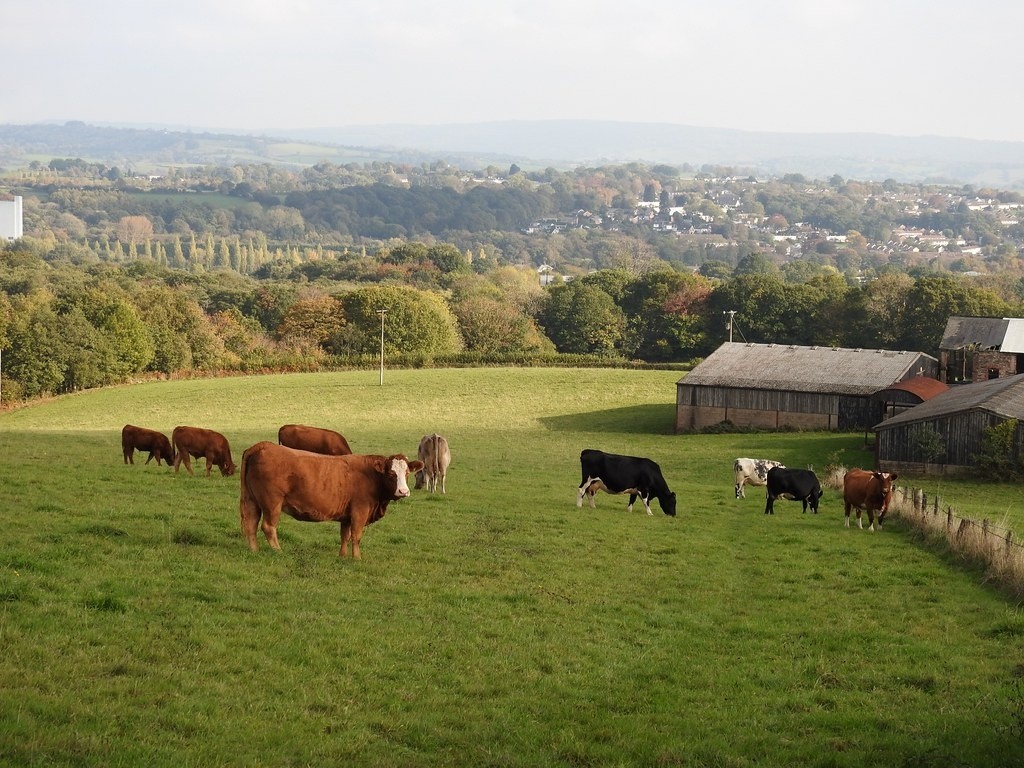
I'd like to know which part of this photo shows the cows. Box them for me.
[842,467,898,534]
[277,425,353,455]
[576,448,677,518]
[733,457,786,499]
[238,441,423,560]
[121,424,177,467]
[764,466,824,515]
[171,426,238,478]
[414,433,452,493]
